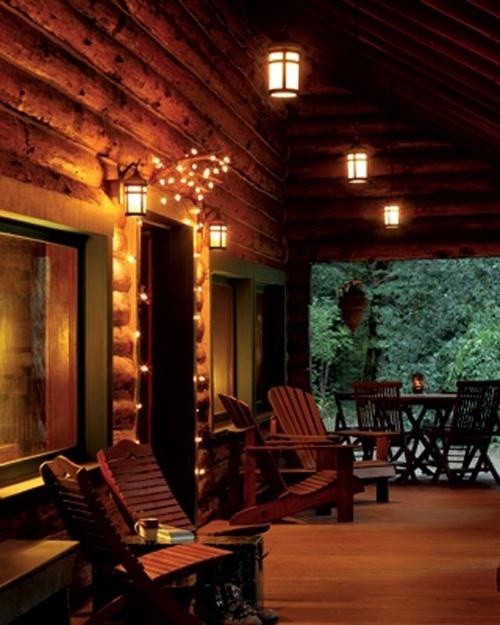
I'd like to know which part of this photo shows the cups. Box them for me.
[134,518,157,542]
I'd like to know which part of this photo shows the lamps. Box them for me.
[346,120,370,184]
[116,157,148,216]
[199,205,228,250]
[265,32,303,96]
[382,194,401,230]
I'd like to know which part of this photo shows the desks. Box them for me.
[0,532,81,625]
[120,528,265,612]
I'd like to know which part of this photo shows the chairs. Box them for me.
[267,376,500,506]
[37,454,234,625]
[215,390,363,526]
[95,439,278,625]
